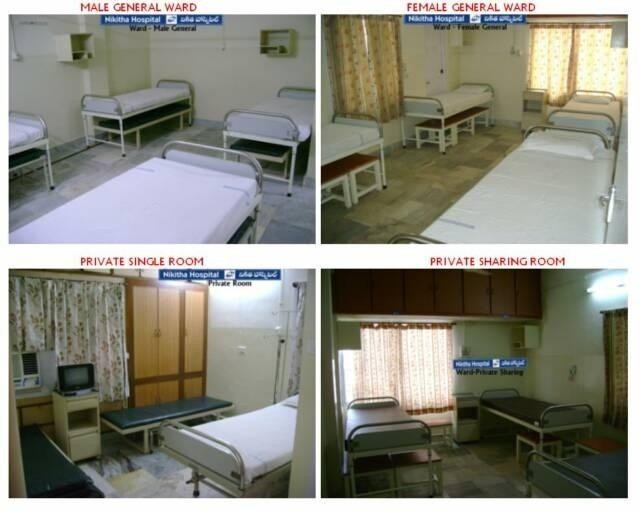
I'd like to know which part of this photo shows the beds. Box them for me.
[344,396,434,498]
[389,82,623,243]
[100,396,234,453]
[158,394,299,498]
[321,111,387,189]
[9,79,316,244]
[480,388,628,497]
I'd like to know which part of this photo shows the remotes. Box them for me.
[64,392,76,396]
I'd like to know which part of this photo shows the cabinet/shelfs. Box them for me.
[56,32,95,62]
[52,391,101,462]
[452,393,480,446]
[125,276,208,407]
[260,28,298,56]
[511,325,540,350]
[335,322,360,349]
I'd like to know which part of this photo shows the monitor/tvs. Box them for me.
[58,363,94,392]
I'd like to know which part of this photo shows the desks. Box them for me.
[16,386,54,441]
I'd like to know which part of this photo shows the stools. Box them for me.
[321,153,383,208]
[343,447,444,498]
